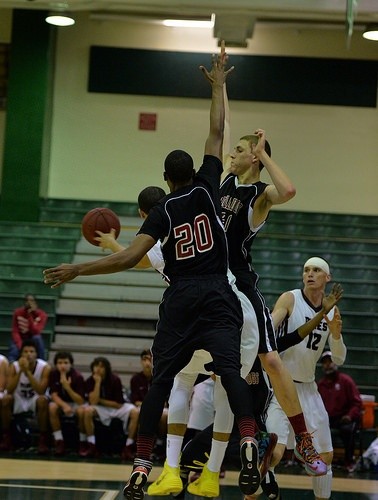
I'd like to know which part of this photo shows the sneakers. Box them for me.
[187,462,219,497]
[220,472,225,478]
[38,432,48,453]
[147,461,182,495]
[261,470,279,500]
[54,440,66,455]
[190,473,199,482]
[0,433,16,450]
[238,437,261,496]
[254,430,279,481]
[79,441,98,460]
[80,440,86,448]
[294,432,327,476]
[347,464,355,472]
[123,466,148,500]
[121,444,135,461]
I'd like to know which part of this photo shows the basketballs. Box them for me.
[81,208,121,246]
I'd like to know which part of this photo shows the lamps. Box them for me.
[362,22,378,41]
[44,10,75,27]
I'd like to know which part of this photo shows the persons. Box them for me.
[84,355,139,462]
[266,257,345,500]
[48,350,87,457]
[179,40,328,477]
[41,53,261,500]
[94,187,260,497]
[8,293,48,363]
[0,354,13,452]
[317,350,366,474]
[128,350,170,461]
[6,340,51,455]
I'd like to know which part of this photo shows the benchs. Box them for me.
[251,211,378,474]
[0,198,171,455]
